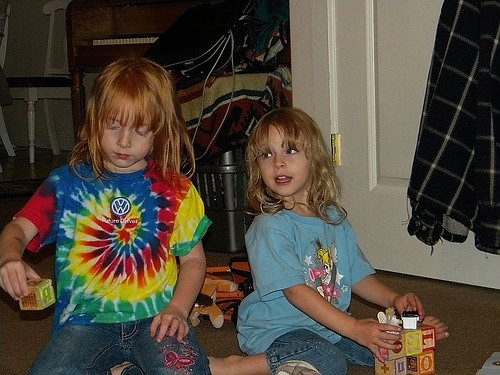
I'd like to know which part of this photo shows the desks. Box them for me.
[6,77,72,164]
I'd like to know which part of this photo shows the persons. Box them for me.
[206,107,449,375]
[0,56,212,375]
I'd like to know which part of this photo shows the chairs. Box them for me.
[42,0,69,155]
[0,4,16,157]
